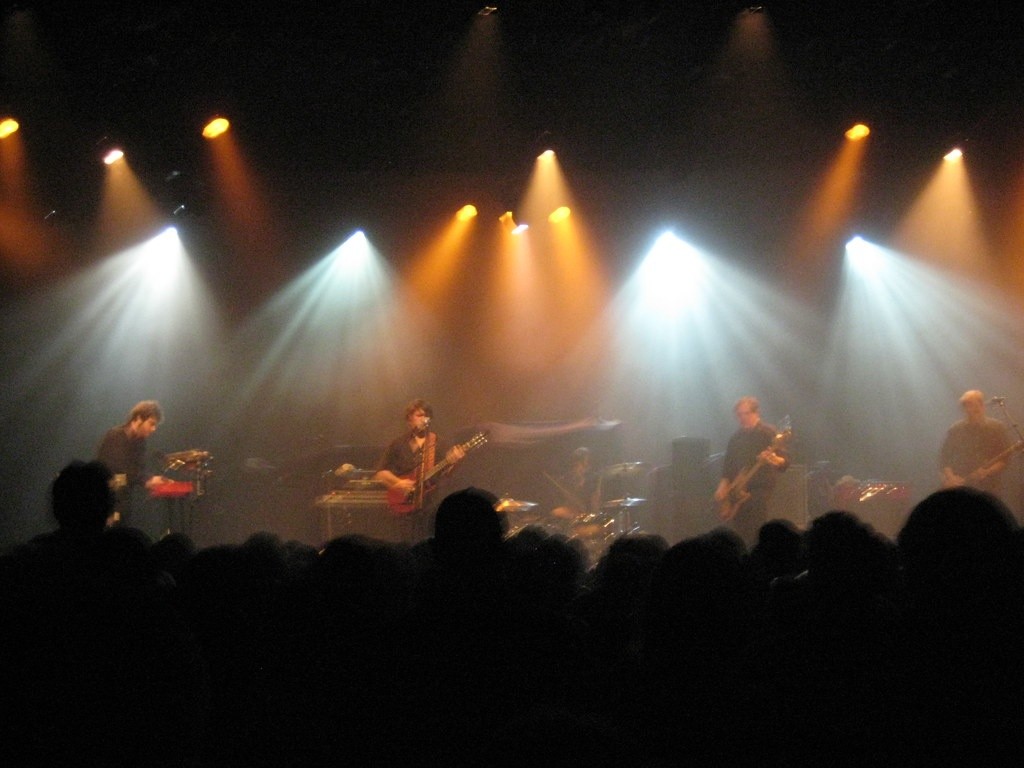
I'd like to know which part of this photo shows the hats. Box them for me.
[959,391,982,405]
[429,489,507,539]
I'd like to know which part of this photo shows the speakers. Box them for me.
[765,464,808,530]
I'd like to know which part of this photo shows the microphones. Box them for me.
[992,396,1005,403]
[422,417,431,423]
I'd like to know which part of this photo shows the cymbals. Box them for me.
[604,497,649,511]
[497,498,539,510]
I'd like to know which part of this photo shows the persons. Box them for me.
[376,400,464,541]
[935,391,1013,491]
[98,400,175,525]
[716,395,792,548]
[0,459,1024,768]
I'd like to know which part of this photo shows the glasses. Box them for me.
[967,409,981,414]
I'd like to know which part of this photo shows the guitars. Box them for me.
[387,429,489,516]
[713,428,792,523]
[940,438,1023,490]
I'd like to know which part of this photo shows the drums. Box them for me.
[571,512,616,539]
